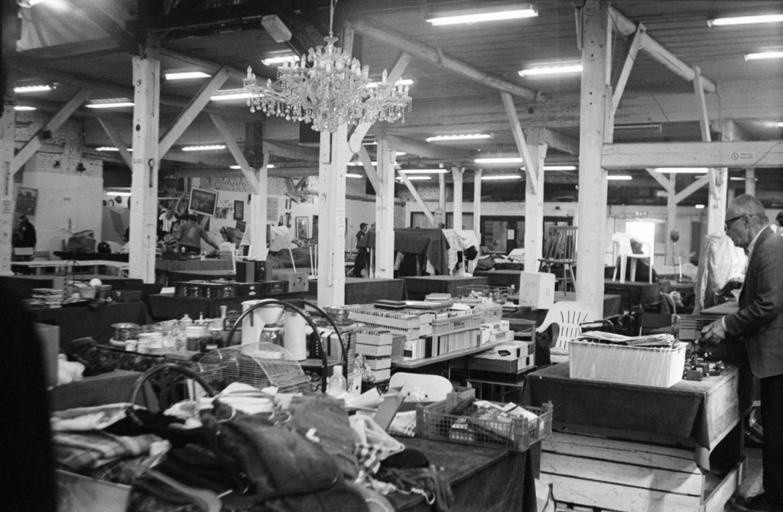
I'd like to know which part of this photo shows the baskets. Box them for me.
[423,399,553,453]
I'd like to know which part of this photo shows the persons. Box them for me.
[617,239,657,283]
[14,214,38,276]
[697,191,783,512]
[176,213,220,254]
[353,221,369,278]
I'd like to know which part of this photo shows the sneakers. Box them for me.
[730,494,765,512]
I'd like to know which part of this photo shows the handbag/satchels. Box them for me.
[125,362,341,499]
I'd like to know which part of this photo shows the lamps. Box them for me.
[240,0,412,134]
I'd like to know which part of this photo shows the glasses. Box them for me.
[724,215,744,230]
